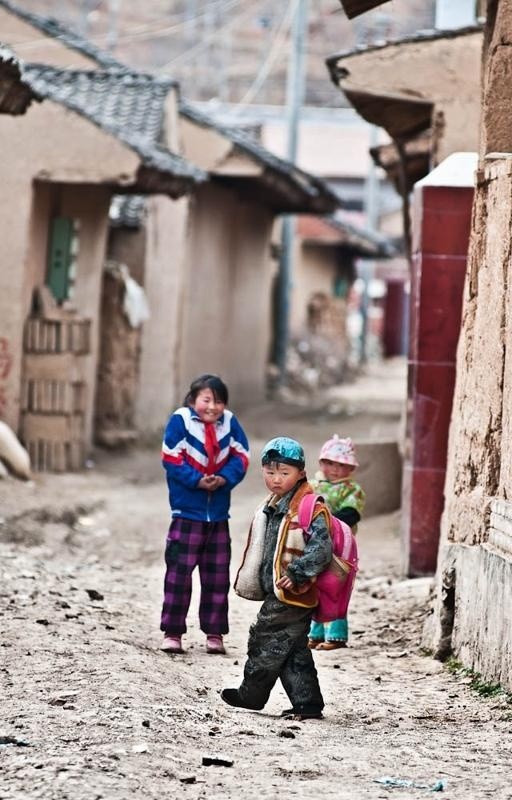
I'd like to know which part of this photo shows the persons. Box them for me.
[218,434,335,720]
[307,433,367,651]
[159,373,250,654]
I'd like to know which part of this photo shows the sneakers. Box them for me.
[220,687,265,710]
[308,639,346,650]
[281,703,323,717]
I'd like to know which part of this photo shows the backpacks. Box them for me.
[297,493,359,624]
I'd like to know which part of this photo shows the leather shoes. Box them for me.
[205,633,226,653]
[161,635,182,652]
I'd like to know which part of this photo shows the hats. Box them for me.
[261,437,306,468]
[318,434,360,467]
